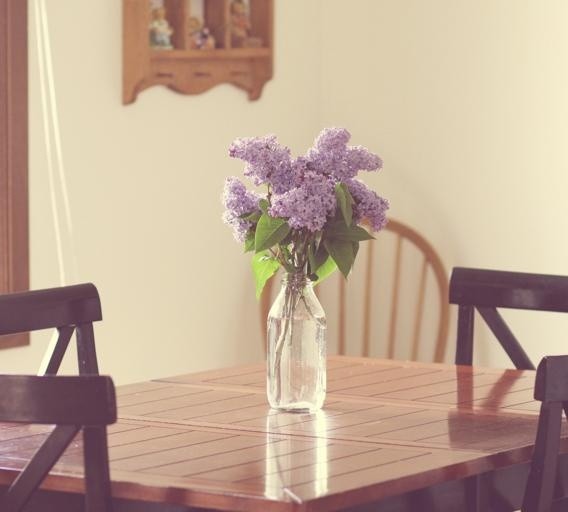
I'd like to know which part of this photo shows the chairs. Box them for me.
[0,283,120,511]
[261,213,449,360]
[447,265,568,512]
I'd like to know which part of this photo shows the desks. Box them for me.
[0,358,565,512]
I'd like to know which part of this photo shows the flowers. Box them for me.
[218,122,390,404]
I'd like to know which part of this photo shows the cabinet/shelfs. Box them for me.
[121,1,273,104]
